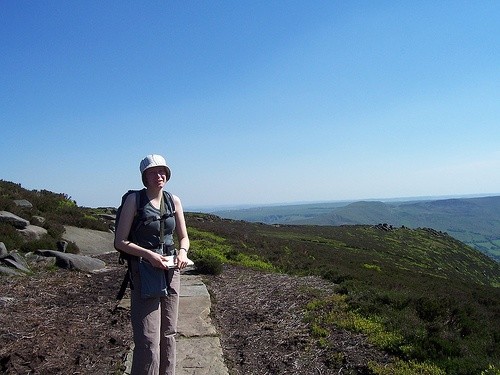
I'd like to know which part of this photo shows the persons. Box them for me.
[114,154,190,375]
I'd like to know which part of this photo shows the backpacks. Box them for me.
[114,190,174,260]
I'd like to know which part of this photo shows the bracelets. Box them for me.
[180,247,188,252]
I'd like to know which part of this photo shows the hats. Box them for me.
[140,155,171,187]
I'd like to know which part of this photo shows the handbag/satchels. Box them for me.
[138,249,168,299]
